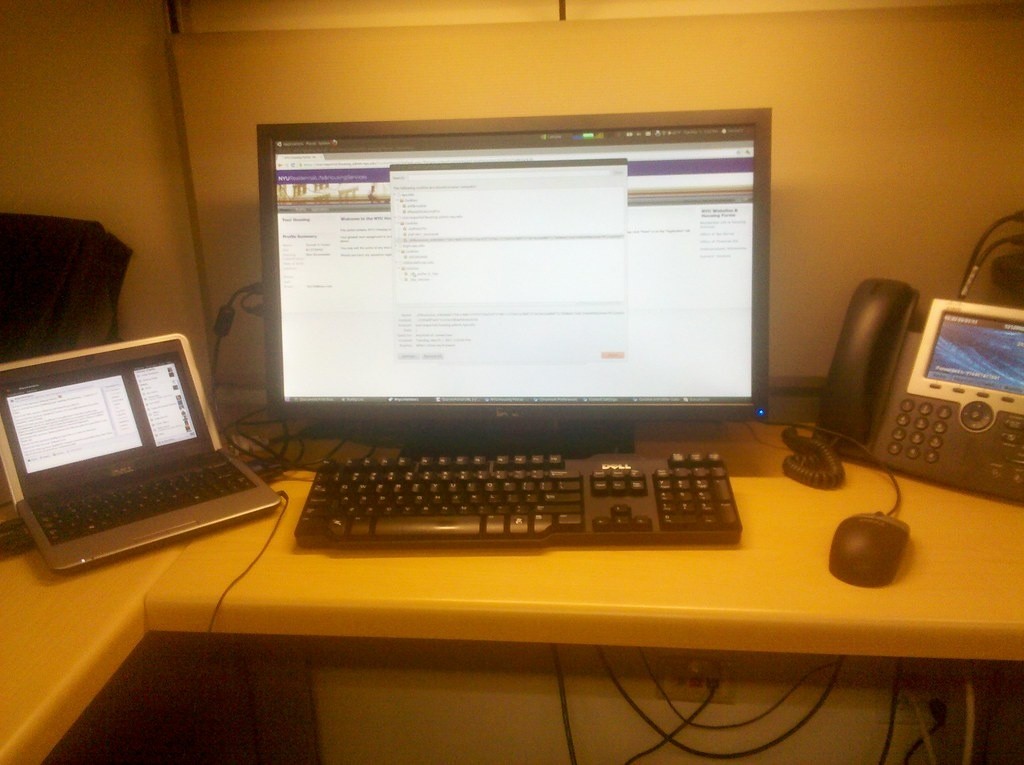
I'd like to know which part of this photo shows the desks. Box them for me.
[0,420,1024,765]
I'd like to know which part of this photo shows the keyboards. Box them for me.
[294,455,740,546]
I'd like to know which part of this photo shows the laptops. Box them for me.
[0,333,281,575]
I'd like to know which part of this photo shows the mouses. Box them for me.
[830,511,909,588]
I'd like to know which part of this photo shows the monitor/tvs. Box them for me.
[256,108,770,463]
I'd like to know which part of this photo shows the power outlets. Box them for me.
[860,674,947,726]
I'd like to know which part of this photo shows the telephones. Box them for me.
[813,274,1024,501]
